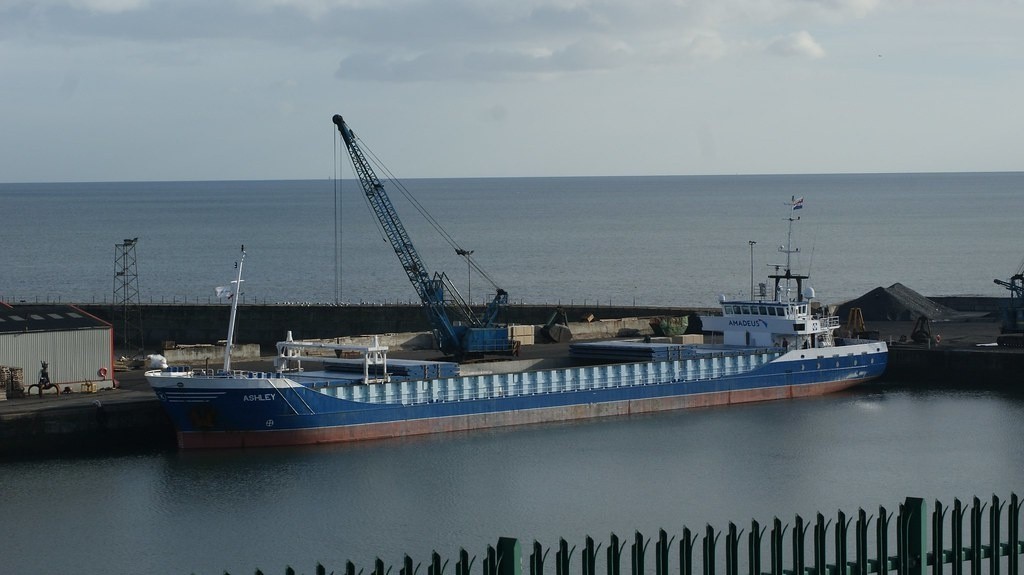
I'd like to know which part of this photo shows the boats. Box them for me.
[143,194,888,450]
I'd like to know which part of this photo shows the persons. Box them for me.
[643,333,651,343]
[783,338,788,353]
[201,369,206,375]
[824,305,829,314]
[804,340,810,349]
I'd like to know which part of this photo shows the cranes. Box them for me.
[333,113,520,361]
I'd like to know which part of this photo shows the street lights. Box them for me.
[748,241,756,301]
[465,250,475,318]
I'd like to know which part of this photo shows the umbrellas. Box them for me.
[848,308,864,339]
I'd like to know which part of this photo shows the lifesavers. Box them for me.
[935,335,941,343]
[99,367,108,377]
